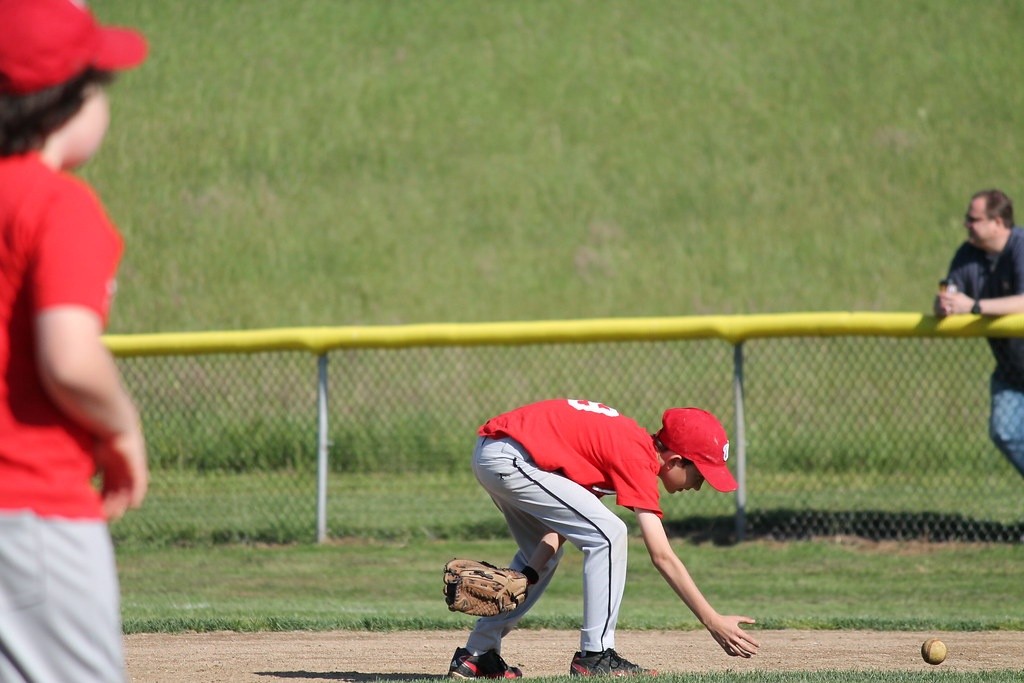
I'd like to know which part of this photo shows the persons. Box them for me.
[443,398,761,680]
[933,189,1024,479]
[0,1,151,683]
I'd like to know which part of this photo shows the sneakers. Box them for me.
[570,648,660,678]
[448,647,522,682]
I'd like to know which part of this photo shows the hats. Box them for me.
[657,407,739,492]
[0,0,149,96]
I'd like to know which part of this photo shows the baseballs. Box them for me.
[920,640,947,665]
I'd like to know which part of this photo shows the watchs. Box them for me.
[972,299,980,314]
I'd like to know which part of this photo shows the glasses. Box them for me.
[965,214,993,223]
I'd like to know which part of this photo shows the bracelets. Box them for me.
[521,566,539,585]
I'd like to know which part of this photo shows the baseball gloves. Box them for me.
[440,558,531,618]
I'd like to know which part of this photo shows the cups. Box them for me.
[939,279,958,311]
